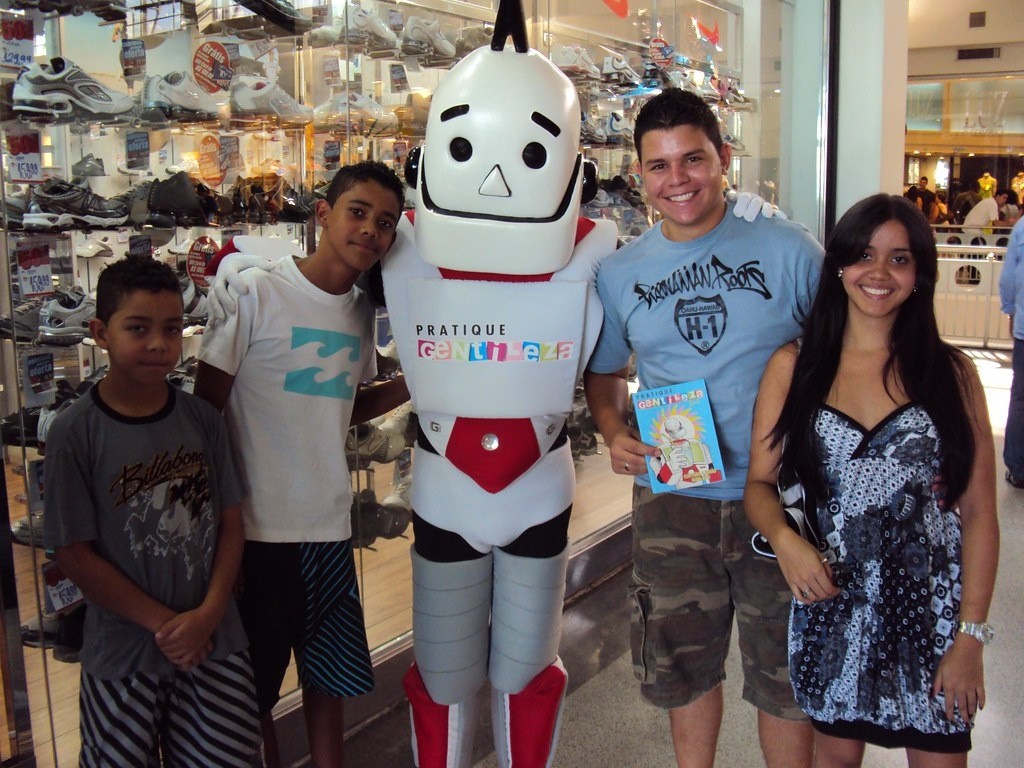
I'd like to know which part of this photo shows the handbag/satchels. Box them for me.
[751,339,820,559]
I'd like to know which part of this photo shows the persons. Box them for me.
[904,177,1024,285]
[978,172,998,233]
[999,214,1024,487]
[1010,173,1024,205]
[202,1,787,767]
[46,256,262,768]
[584,87,959,768]
[743,192,1000,768]
[195,161,405,768]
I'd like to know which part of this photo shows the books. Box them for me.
[632,379,726,493]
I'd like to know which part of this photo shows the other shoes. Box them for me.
[1004,470,1022,488]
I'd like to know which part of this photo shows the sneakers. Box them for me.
[0,0,755,669]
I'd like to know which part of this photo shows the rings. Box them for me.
[625,463,629,471]
[804,590,811,596]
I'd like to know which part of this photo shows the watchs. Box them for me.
[957,622,993,645]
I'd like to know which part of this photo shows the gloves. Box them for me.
[726,189,787,222]
[207,252,274,327]
